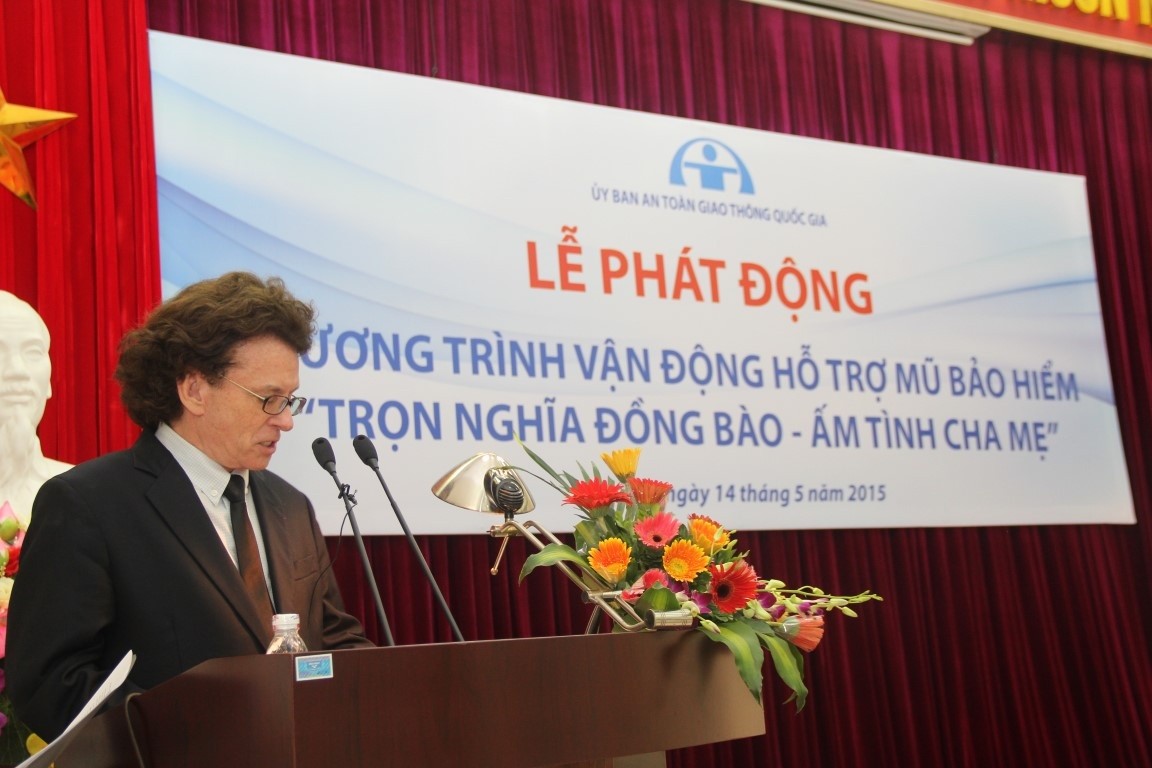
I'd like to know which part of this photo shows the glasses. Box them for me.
[215,374,308,417]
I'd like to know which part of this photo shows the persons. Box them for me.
[0,289,75,531]
[4,270,377,743]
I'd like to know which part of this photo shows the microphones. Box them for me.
[312,436,397,648]
[353,434,465,641]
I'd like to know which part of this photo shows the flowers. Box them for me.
[501,429,887,718]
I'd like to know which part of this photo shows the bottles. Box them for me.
[265,614,308,654]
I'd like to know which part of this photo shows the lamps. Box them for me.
[429,452,651,635]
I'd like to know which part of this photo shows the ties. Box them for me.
[222,473,278,645]
